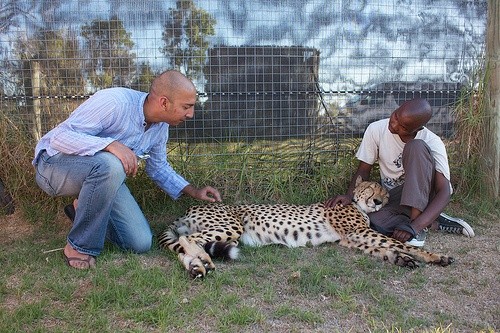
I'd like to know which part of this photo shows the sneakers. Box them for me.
[436,213,474,237]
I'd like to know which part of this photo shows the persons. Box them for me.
[323,98,475,243]
[31,69,222,271]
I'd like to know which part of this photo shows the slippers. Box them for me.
[61,248,96,270]
[64,204,77,221]
